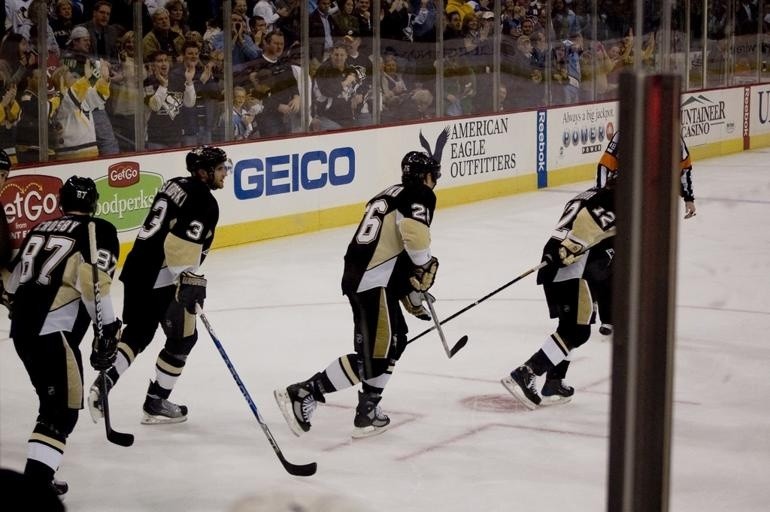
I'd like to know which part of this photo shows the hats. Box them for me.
[343,28,361,42]
[482,11,494,19]
[65,26,90,45]
[517,35,530,45]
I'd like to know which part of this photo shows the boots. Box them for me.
[354,390,391,427]
[142,379,188,419]
[286,371,327,433]
[89,364,121,419]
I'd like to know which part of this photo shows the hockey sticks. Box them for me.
[88,221,134,446]
[194,302,316,476]
[424,292,467,357]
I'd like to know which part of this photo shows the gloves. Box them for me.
[554,238,585,268]
[410,253,438,294]
[175,271,208,316]
[90,317,122,371]
[401,289,436,323]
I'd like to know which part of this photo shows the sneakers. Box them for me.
[598,313,613,338]
[542,379,575,398]
[510,365,542,407]
[47,477,69,496]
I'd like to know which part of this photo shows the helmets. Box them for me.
[60,175,98,215]
[185,144,227,189]
[399,151,440,190]
[1,147,13,174]
